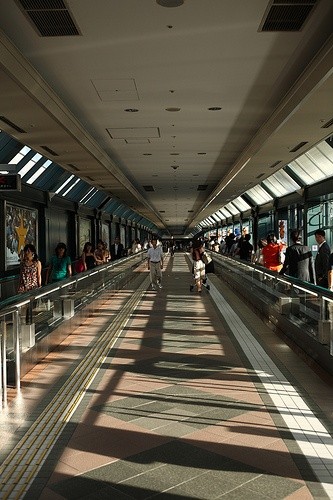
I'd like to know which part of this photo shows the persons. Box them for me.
[279,229,315,307]
[94,242,111,266]
[218,233,253,262]
[111,237,123,261]
[18,244,41,325]
[147,238,164,289]
[131,237,176,257]
[192,240,206,292]
[253,231,283,280]
[327,252,333,320]
[314,229,331,320]
[45,243,73,302]
[174,237,219,253]
[81,242,103,289]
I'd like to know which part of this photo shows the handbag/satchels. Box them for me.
[201,252,212,264]
[279,252,286,264]
[74,258,85,273]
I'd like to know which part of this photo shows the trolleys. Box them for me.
[189,261,211,292]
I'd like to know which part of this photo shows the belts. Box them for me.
[150,261,160,263]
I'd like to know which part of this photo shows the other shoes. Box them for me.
[152,287,157,290]
[197,287,203,293]
[158,285,164,288]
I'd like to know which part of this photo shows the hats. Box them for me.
[192,240,204,248]
